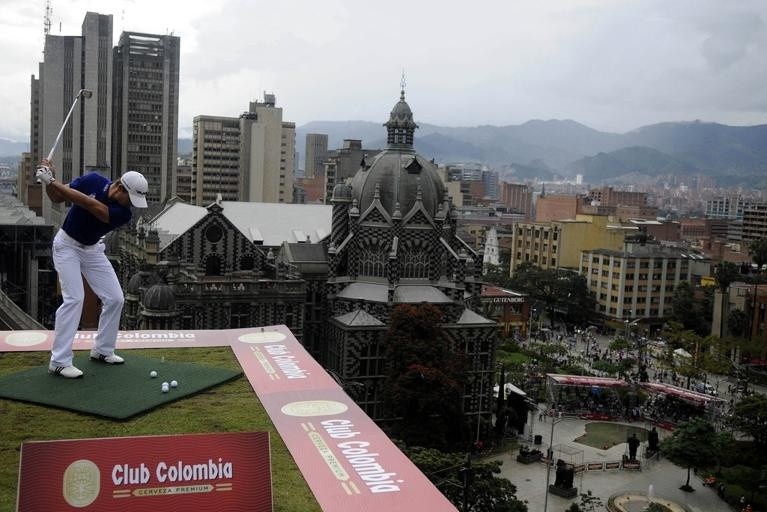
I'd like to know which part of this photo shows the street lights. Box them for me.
[581,325,598,376]
[624,309,637,339]
[528,306,536,345]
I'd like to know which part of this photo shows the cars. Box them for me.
[699,383,716,395]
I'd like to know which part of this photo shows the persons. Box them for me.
[515,311,754,430]
[645,427,658,451]
[627,433,640,460]
[33,157,150,381]
[702,474,716,486]
[716,482,725,499]
[740,503,751,512]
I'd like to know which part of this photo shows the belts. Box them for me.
[58,228,86,248]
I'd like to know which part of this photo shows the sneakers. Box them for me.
[89,348,124,365]
[47,362,84,378]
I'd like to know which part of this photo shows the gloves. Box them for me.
[35,157,55,187]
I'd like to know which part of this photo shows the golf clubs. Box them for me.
[37,89,92,184]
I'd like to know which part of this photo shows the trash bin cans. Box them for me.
[535,435,542,444]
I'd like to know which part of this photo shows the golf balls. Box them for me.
[151,371,157,377]
[171,381,177,386]
[162,386,168,392]
[162,382,169,386]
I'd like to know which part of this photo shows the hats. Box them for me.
[122,170,149,211]
